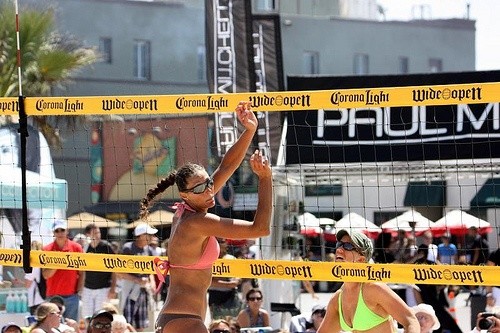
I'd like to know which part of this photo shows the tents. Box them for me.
[0,164,68,210]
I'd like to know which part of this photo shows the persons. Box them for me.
[316,229,420,333]
[0,220,270,333]
[282,226,500,333]
[139,101,272,333]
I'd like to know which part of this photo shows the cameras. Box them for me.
[479,313,497,330]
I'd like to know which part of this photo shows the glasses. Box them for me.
[213,329,230,333]
[55,228,65,232]
[336,240,363,255]
[249,296,262,301]
[182,176,214,194]
[93,323,112,329]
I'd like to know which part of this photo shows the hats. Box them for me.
[46,295,64,305]
[312,304,326,313]
[90,309,113,321]
[1,321,22,332]
[336,228,374,262]
[53,218,68,231]
[134,223,158,236]
[410,303,441,330]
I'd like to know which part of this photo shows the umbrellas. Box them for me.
[297,209,493,240]
[67,210,176,227]
